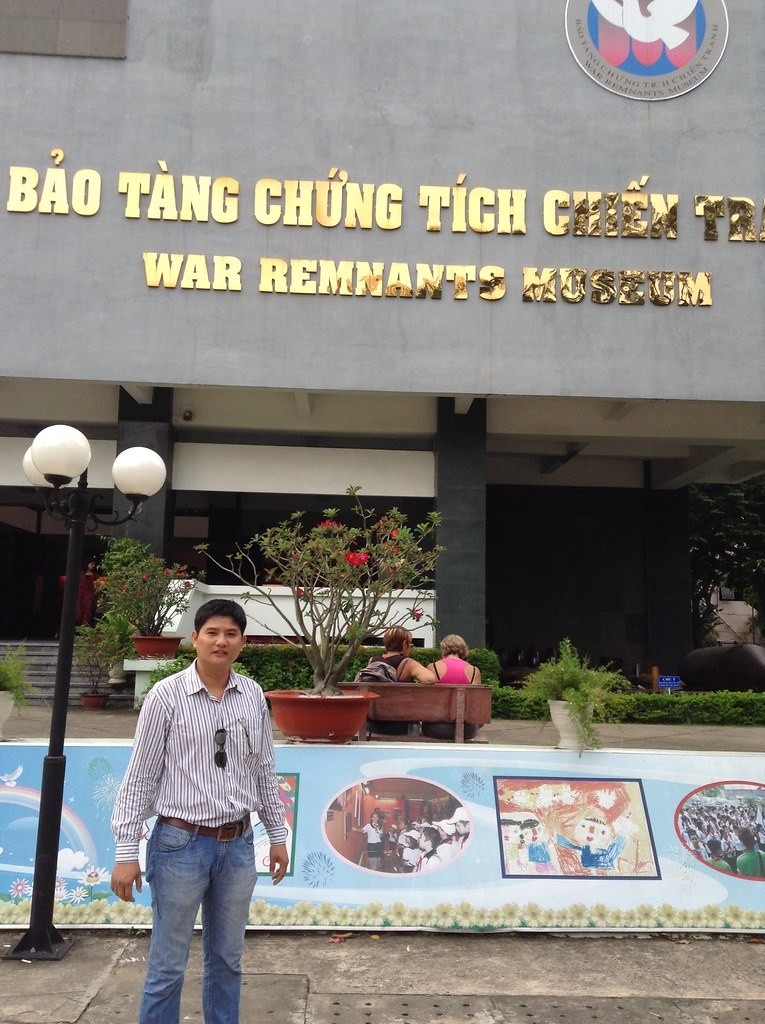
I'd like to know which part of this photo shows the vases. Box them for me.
[264,689,380,745]
[129,635,186,659]
[78,691,112,710]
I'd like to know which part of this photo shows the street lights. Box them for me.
[2,425,166,961]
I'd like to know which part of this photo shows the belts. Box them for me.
[158,813,250,841]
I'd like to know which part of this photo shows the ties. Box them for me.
[374,824,377,831]
[694,850,701,856]
[725,840,731,849]
[716,826,719,831]
[416,856,423,872]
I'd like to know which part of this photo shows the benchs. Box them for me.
[337,681,493,744]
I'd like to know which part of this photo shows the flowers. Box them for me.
[194,482,449,697]
[54,625,131,695]
[91,533,207,637]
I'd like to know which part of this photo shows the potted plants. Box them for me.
[517,637,632,758]
[92,611,135,684]
[0,637,52,741]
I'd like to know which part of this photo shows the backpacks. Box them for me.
[355,654,404,681]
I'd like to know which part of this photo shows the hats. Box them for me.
[403,830,420,840]
[447,807,470,824]
[432,820,456,835]
[412,821,438,830]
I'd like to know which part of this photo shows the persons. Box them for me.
[110,598,288,1024]
[679,785,765,879]
[350,807,473,873]
[367,625,437,734]
[421,634,484,739]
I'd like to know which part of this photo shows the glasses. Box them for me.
[215,729,226,770]
